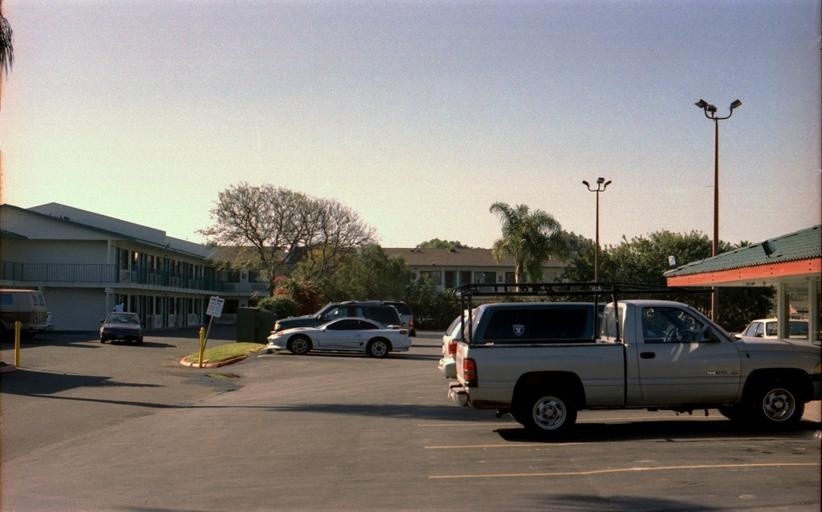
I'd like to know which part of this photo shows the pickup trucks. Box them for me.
[447,297,821,438]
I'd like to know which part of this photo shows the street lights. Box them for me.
[582,177,613,295]
[695,99,742,324]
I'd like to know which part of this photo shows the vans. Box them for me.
[0,288,51,343]
[460,301,610,346]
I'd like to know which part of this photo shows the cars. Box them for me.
[438,308,477,377]
[734,319,811,341]
[267,316,411,357]
[341,300,414,335]
[99,310,146,345]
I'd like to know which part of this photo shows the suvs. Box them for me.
[274,300,402,333]
